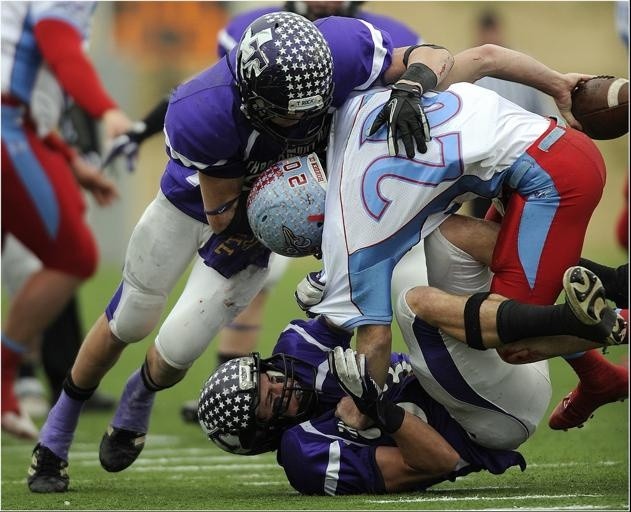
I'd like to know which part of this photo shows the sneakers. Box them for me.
[99,380,155,473]
[562,265,627,345]
[549,366,629,431]
[27,408,78,493]
[182,397,200,423]
[2,393,40,440]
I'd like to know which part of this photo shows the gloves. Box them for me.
[327,346,382,414]
[295,270,327,310]
[368,83,432,160]
[102,133,138,175]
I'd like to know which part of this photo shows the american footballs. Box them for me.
[571,77,629,140]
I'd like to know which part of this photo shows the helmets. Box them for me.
[197,355,286,455]
[248,153,328,256]
[236,12,335,128]
